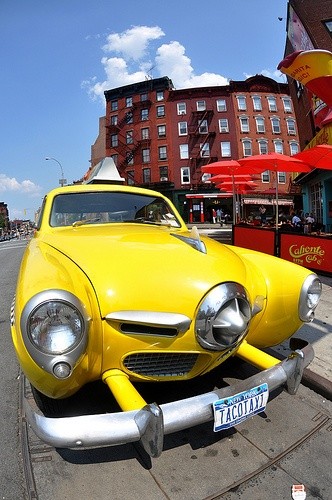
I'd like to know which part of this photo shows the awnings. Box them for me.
[243,199,293,206]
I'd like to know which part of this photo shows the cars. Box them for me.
[9,156,323,458]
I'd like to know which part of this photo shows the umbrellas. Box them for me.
[236,152,313,231]
[207,174,262,220]
[200,160,261,223]
[258,186,285,211]
[290,145,332,170]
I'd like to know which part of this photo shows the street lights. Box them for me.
[46,156,64,187]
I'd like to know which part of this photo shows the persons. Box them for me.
[205,207,223,223]
[268,211,315,227]
[259,204,267,221]
[16,228,27,240]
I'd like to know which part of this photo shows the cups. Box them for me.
[304,225,308,234]
[317,229,321,236]
